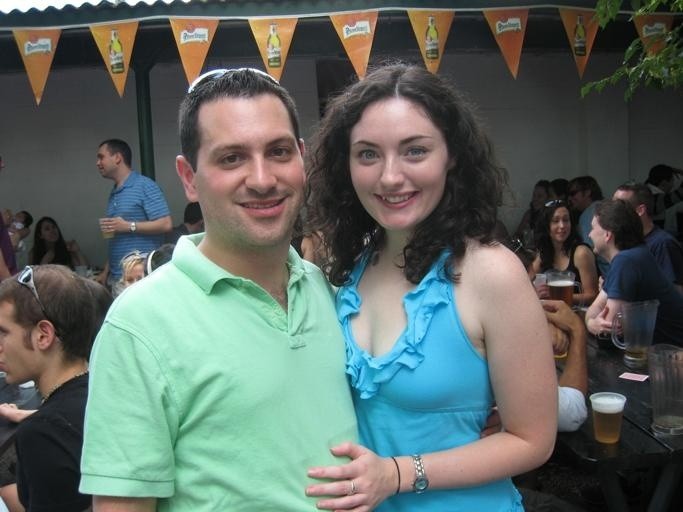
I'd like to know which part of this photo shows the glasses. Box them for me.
[188,67,280,93]
[545,200,564,207]
[18,266,60,337]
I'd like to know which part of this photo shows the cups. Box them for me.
[521,229,534,248]
[647,343,683,436]
[74,265,88,277]
[97,217,113,240]
[534,274,546,289]
[547,270,582,315]
[589,391,627,444]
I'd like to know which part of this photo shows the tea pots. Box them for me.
[609,298,661,369]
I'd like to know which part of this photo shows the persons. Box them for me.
[499,164,683,398]
[0,137,204,512]
[79,65,558,511]
